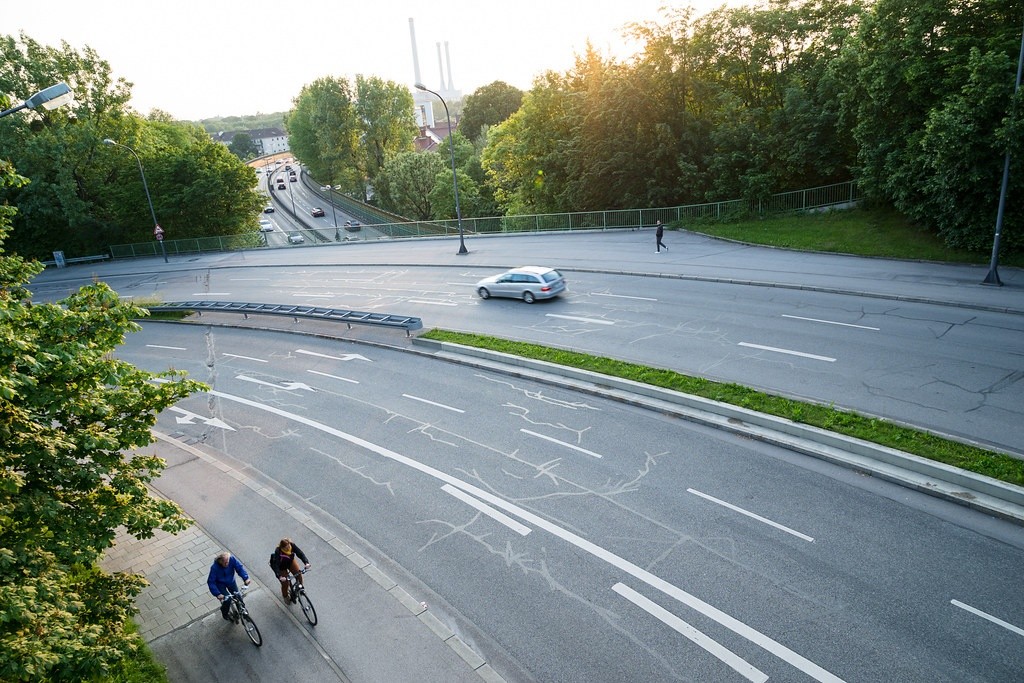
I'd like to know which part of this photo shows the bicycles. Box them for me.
[222,585,262,647]
[280,564,318,626]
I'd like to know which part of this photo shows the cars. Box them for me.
[290,176,297,182]
[288,232,305,245]
[311,207,325,218]
[277,177,284,183]
[475,266,567,304]
[278,183,286,190]
[344,221,362,232]
[259,220,273,232]
[256,159,296,176]
[264,203,274,213]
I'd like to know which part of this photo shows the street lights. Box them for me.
[104,139,169,264]
[320,185,341,242]
[267,158,273,183]
[414,82,468,254]
[287,169,297,222]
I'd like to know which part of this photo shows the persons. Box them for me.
[655,220,668,253]
[271,538,311,605]
[207,553,250,620]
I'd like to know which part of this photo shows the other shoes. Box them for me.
[284,596,291,605]
[221,607,230,620]
